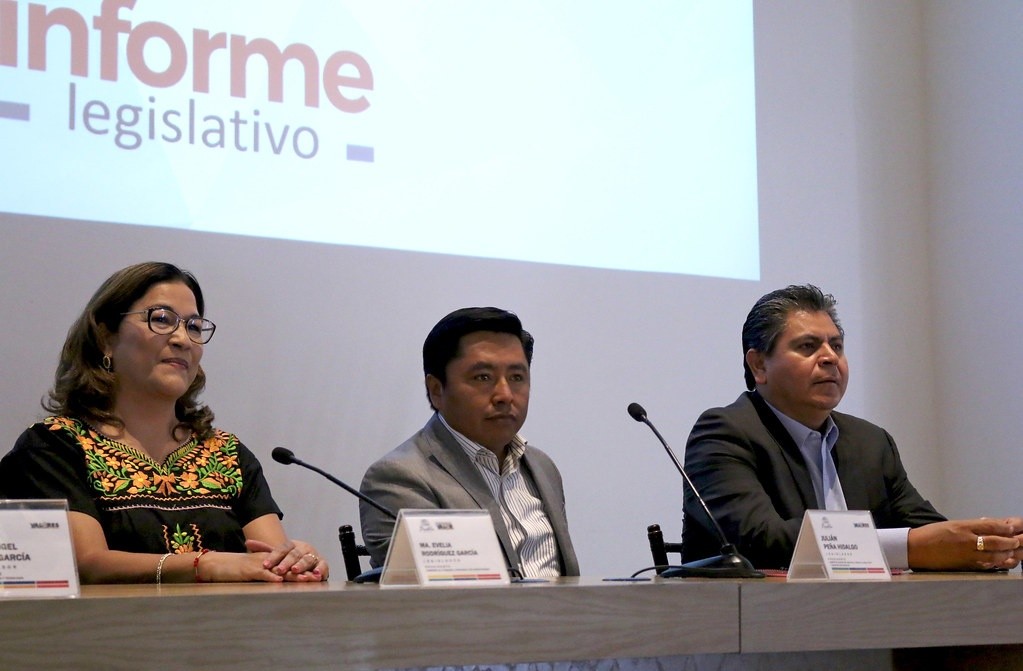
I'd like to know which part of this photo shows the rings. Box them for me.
[976,535,985,551]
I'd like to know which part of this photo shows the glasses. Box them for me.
[116,309,217,345]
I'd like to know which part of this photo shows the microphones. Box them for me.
[272,446,397,522]
[628,403,765,578]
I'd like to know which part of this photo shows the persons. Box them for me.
[1,258,331,588]
[680,286,1023,582]
[358,305,584,583]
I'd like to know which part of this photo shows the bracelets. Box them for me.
[155,551,175,584]
[192,548,213,584]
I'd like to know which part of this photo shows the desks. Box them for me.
[0,568,1023,671]
